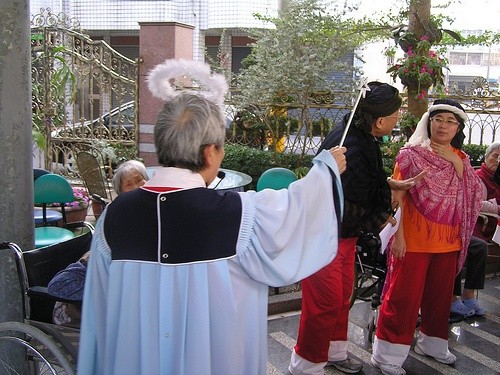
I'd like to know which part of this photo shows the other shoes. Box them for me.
[448,311,464,323]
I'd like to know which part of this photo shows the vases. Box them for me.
[50,207,89,227]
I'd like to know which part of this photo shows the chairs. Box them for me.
[348,231,384,316]
[34,149,117,248]
[257,167,298,191]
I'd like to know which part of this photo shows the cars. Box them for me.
[50,99,264,176]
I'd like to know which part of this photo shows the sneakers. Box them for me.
[371,357,406,375]
[414,344,456,365]
[325,355,363,373]
[450,299,487,320]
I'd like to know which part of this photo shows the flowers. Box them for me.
[387,19,461,100]
[51,188,89,206]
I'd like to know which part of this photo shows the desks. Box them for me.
[145,166,252,194]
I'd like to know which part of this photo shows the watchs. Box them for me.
[385,175,391,184]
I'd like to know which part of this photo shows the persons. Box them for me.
[445,143,500,316]
[287,81,432,375]
[371,97,488,375]
[48,160,151,302]
[74,88,348,375]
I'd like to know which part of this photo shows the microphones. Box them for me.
[214,171,225,190]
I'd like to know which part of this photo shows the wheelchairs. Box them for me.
[0,221,95,374]
[93,193,112,210]
[349,203,487,341]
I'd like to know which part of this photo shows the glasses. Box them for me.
[429,117,460,125]
